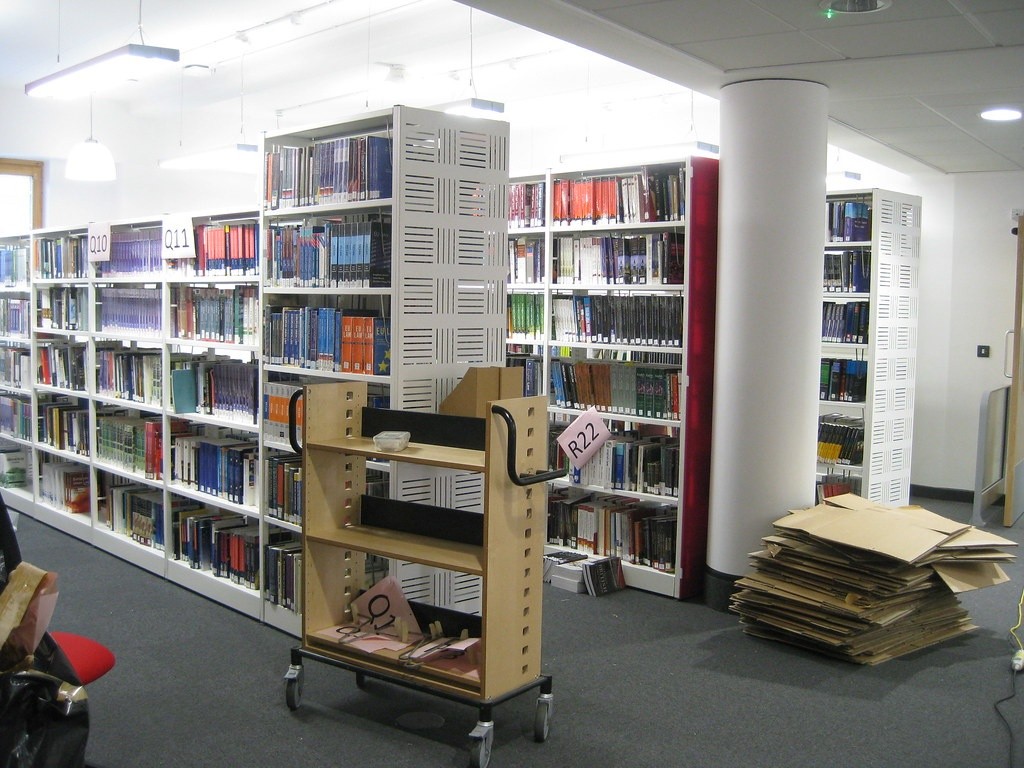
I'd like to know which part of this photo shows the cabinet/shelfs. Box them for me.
[814,189,921,507]
[262,105,511,641]
[282,379,567,768]
[0,205,264,622]
[509,155,720,602]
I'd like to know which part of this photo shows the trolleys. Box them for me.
[284,374,575,768]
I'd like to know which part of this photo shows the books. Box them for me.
[506,168,685,598]
[0,136,392,613]
[815,200,872,505]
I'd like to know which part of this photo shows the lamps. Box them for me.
[24,44,861,182]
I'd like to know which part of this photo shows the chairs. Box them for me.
[0,492,116,687]
